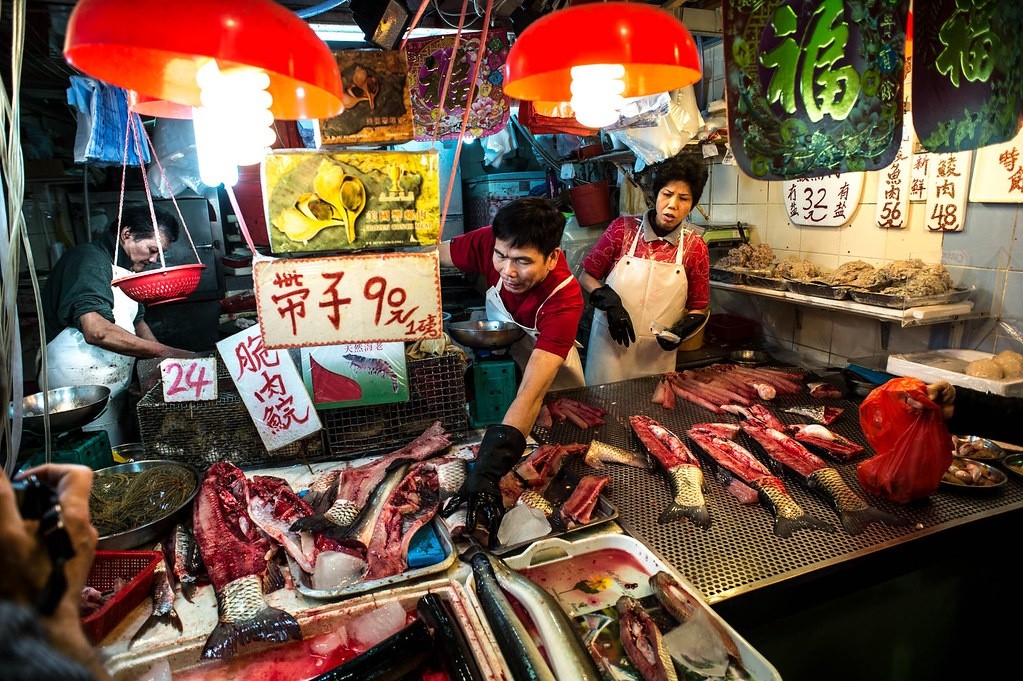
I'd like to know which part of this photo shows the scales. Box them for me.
[10,384,114,475]
[845,351,991,432]
[445,322,520,426]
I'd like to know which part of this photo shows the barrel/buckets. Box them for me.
[676,308,711,351]
[570,179,611,228]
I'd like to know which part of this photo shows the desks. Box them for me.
[708,279,996,350]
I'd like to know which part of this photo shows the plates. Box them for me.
[84,456,199,552]
[725,349,773,366]
[1001,453,1023,477]
[938,458,1008,491]
[949,436,1006,460]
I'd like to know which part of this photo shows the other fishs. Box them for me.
[129,351,1023,680]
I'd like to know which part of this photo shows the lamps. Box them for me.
[62,0,345,189]
[348,0,414,53]
[503,0,703,128]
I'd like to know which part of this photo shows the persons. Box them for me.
[579,152,711,388]
[36,203,181,448]
[0,463,113,681]
[417,196,585,550]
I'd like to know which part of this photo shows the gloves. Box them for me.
[590,285,637,348]
[444,423,525,550]
[652,314,706,350]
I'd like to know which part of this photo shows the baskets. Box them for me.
[80,550,164,646]
[110,265,204,306]
